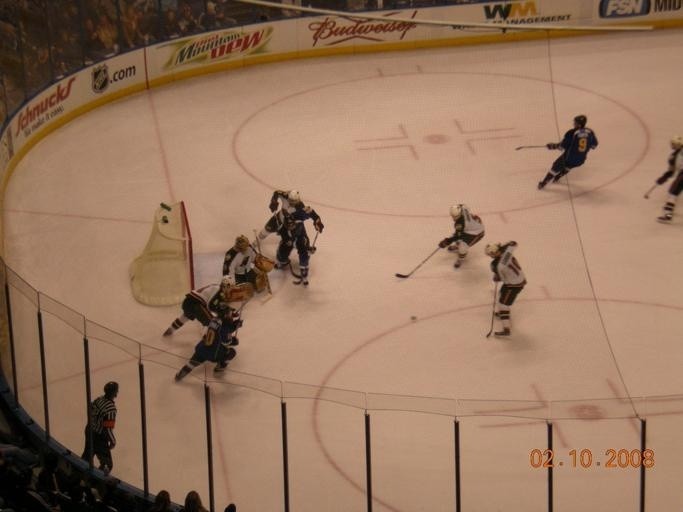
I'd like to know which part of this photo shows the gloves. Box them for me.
[269,202,279,213]
[547,142,556,150]
[313,217,324,233]
[438,237,452,248]
[657,176,666,185]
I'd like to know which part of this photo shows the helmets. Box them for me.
[104,381,118,394]
[486,241,501,255]
[449,204,462,221]
[217,304,230,317]
[220,276,236,289]
[235,234,249,250]
[289,189,301,204]
[672,135,682,147]
[575,116,587,128]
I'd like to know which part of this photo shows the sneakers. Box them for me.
[538,182,546,190]
[454,259,463,268]
[274,263,288,269]
[658,214,673,220]
[554,176,560,182]
[302,271,307,284]
[175,370,187,381]
[163,327,175,337]
[216,362,228,371]
[495,327,511,335]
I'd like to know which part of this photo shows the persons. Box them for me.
[76,380,120,475]
[438,203,485,268]
[537,114,599,189]
[156,187,324,383]
[0,431,241,511]
[654,136,682,221]
[482,239,528,336]
[0,1,382,130]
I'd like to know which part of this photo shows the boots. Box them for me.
[448,243,458,250]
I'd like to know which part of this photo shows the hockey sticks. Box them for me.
[485,281,498,338]
[232,265,253,339]
[644,184,659,198]
[396,246,441,278]
[515,145,547,151]
[293,230,320,285]
[253,228,272,303]
